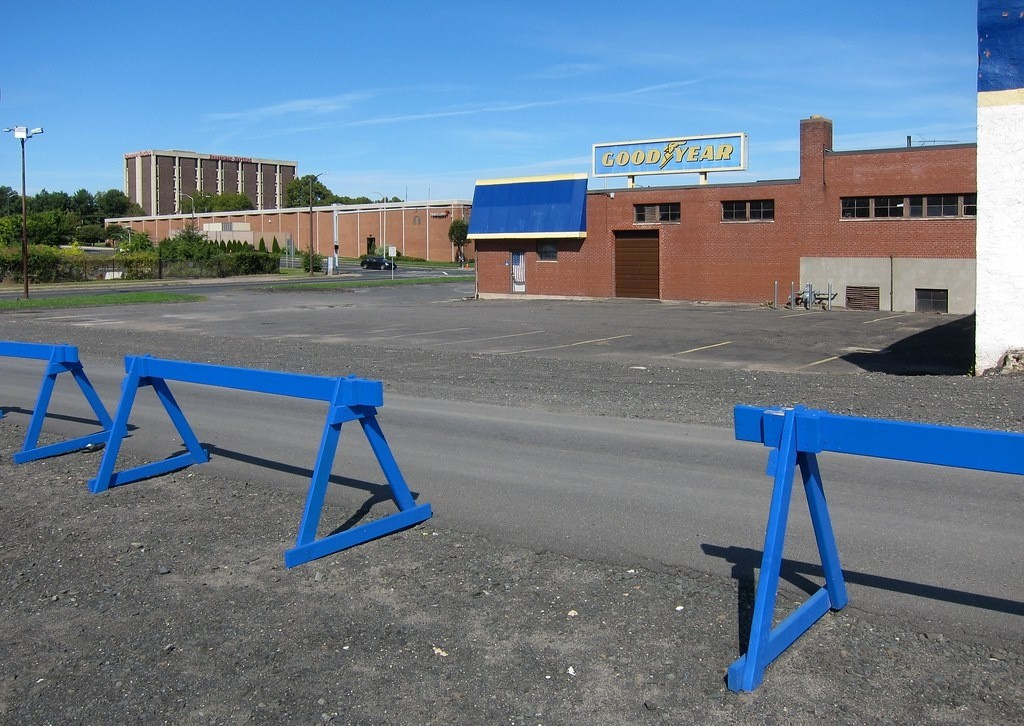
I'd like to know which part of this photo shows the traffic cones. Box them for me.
[465,263,470,269]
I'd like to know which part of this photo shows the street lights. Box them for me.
[308,170,329,276]
[3,126,44,299]
[179,196,210,234]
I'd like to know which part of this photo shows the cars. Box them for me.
[360,256,398,271]
